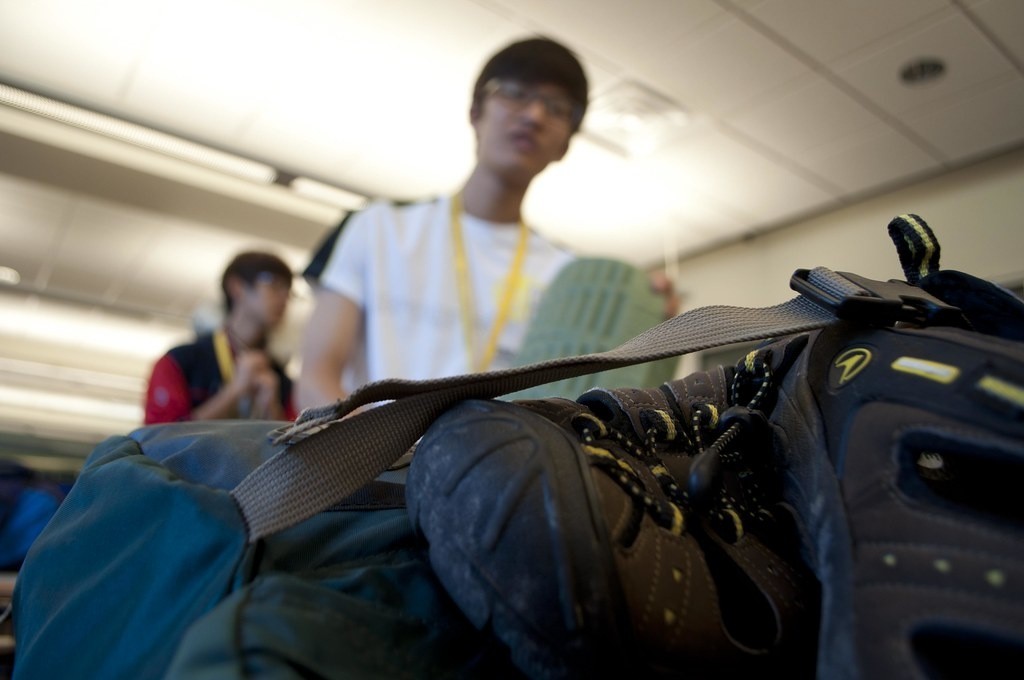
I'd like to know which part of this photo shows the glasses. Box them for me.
[486,81,578,124]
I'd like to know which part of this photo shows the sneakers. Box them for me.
[768,214,1024,680]
[403,330,817,680]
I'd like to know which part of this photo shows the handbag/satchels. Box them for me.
[0,271,963,680]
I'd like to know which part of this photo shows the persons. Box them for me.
[291,38,680,415]
[144,253,298,425]
